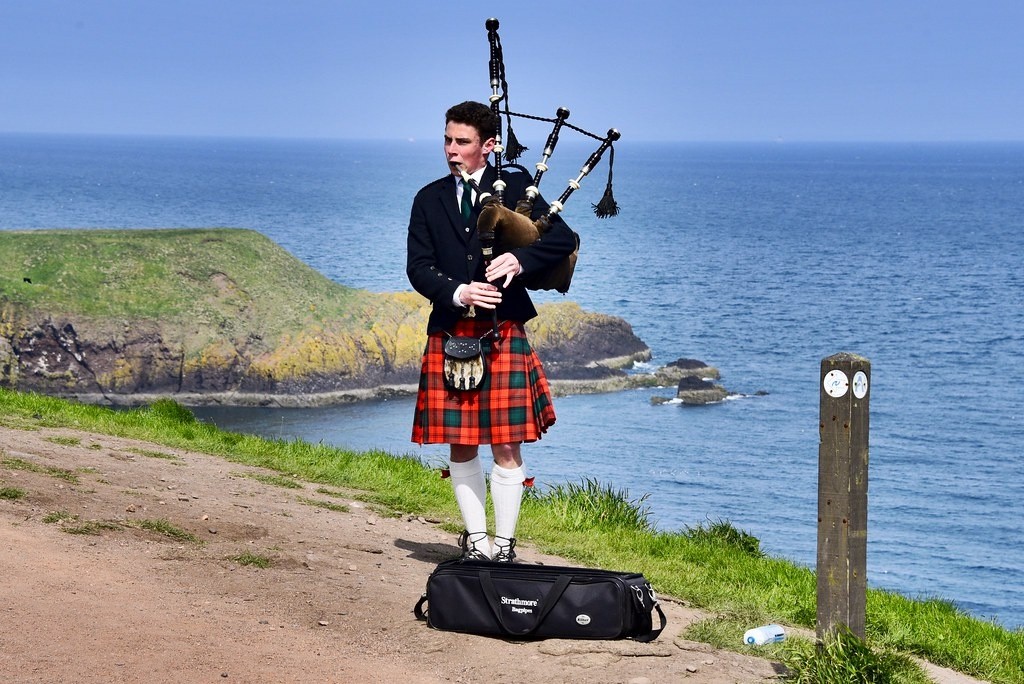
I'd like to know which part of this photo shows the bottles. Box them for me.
[743,624,786,646]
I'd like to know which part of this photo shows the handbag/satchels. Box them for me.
[443,336,487,392]
[415,553,668,645]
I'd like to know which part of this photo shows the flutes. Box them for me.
[453,17,621,342]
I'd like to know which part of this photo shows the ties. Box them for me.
[459,178,472,224]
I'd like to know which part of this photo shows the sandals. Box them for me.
[457,530,491,562]
[491,535,517,563]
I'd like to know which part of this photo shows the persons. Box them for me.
[407,101,576,559]
